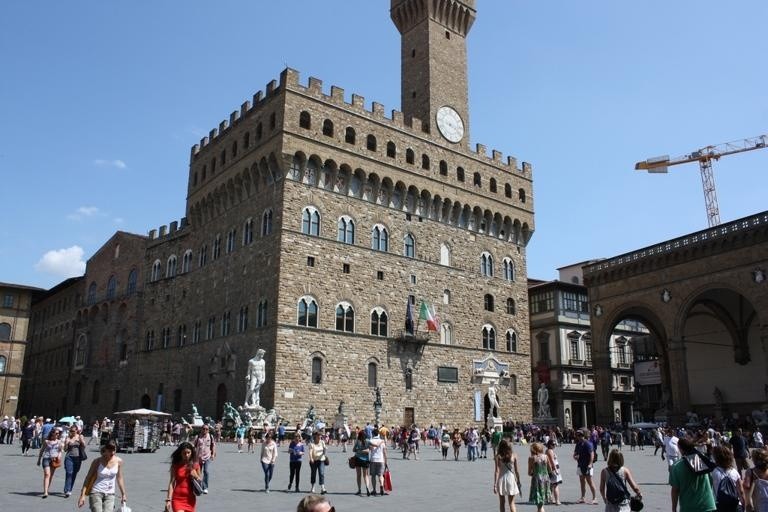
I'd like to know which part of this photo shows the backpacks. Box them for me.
[605,466,630,505]
[746,468,768,512]
[715,466,739,512]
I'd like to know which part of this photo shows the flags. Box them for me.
[404,295,440,335]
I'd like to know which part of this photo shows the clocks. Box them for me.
[435,105,465,144]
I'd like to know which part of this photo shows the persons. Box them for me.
[488,379,500,414]
[223,400,239,421]
[537,382,549,417]
[565,409,570,426]
[615,409,620,423]
[243,348,266,407]
[1,417,768,512]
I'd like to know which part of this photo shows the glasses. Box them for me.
[328,507,335,512]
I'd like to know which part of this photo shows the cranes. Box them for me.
[634,133,768,228]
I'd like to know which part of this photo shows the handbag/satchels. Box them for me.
[117,500,132,512]
[349,457,356,468]
[324,456,329,465]
[51,457,62,468]
[384,465,392,491]
[79,434,88,460]
[189,460,203,496]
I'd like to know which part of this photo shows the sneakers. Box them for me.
[203,489,208,494]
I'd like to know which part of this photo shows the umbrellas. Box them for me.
[113,407,174,419]
